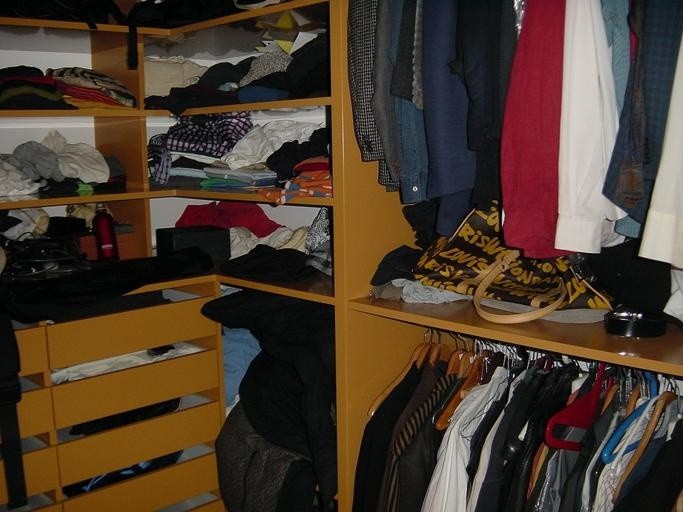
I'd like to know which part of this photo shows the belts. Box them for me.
[604,304,667,338]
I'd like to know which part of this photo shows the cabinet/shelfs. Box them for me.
[0,0,343,512]
[339,0,683,510]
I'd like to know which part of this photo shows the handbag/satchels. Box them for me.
[0,233,78,280]
[412,199,612,311]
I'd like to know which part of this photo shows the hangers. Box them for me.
[367,327,683,502]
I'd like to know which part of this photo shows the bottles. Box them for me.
[92,204,119,261]
